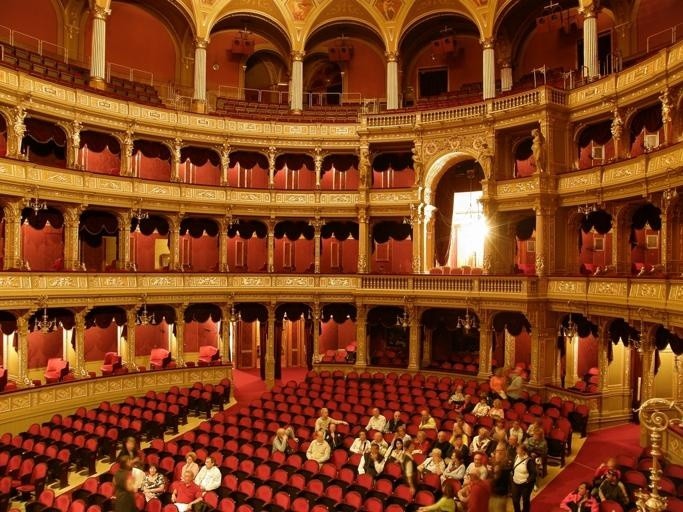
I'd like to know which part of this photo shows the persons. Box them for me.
[559,457,630,512]
[416,364,549,512]
[305,408,349,469]
[349,406,439,481]
[113,436,223,512]
[272,427,300,454]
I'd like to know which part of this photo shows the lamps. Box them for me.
[577,205,597,221]
[396,295,416,334]
[25,184,50,216]
[136,298,157,329]
[455,296,476,335]
[225,303,242,326]
[661,167,679,203]
[34,303,58,337]
[221,207,240,230]
[307,208,326,229]
[626,307,658,361]
[130,198,150,226]
[557,300,580,344]
[402,206,421,230]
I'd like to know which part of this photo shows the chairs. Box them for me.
[0,42,167,110]
[430,265,483,276]
